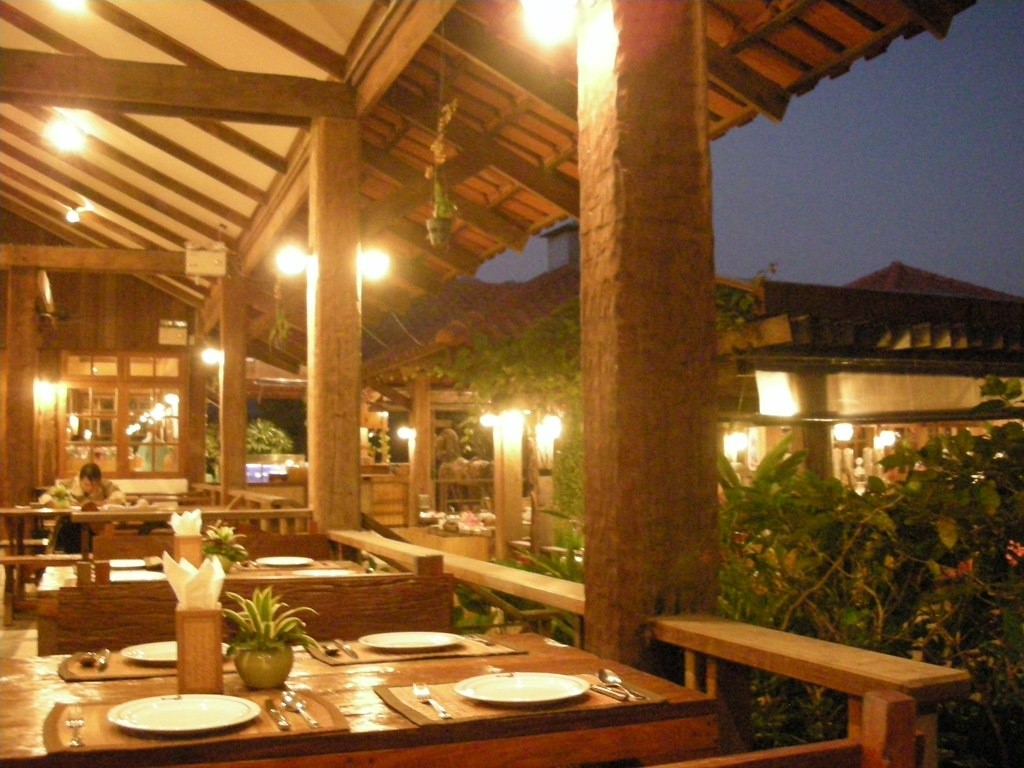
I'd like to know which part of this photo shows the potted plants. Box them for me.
[219,585,325,692]
[48,484,78,510]
[201,524,252,574]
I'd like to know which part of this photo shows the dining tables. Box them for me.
[0,494,381,653]
[0,632,718,768]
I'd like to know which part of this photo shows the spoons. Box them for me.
[597,666,646,700]
[322,645,338,656]
[281,690,319,729]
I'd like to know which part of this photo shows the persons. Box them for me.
[38,463,129,555]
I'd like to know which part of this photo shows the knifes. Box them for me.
[335,639,359,659]
[264,698,291,730]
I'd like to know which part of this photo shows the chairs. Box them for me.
[57,573,454,657]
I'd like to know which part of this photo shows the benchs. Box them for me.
[93,533,334,561]
[0,538,50,589]
[41,519,56,543]
[0,553,94,626]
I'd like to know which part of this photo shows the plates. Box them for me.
[359,632,465,650]
[108,694,261,735]
[292,569,356,577]
[255,556,315,566]
[454,672,591,706]
[120,640,230,665]
[109,559,145,568]
[110,571,166,581]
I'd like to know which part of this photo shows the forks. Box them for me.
[412,683,452,719]
[67,705,85,747]
